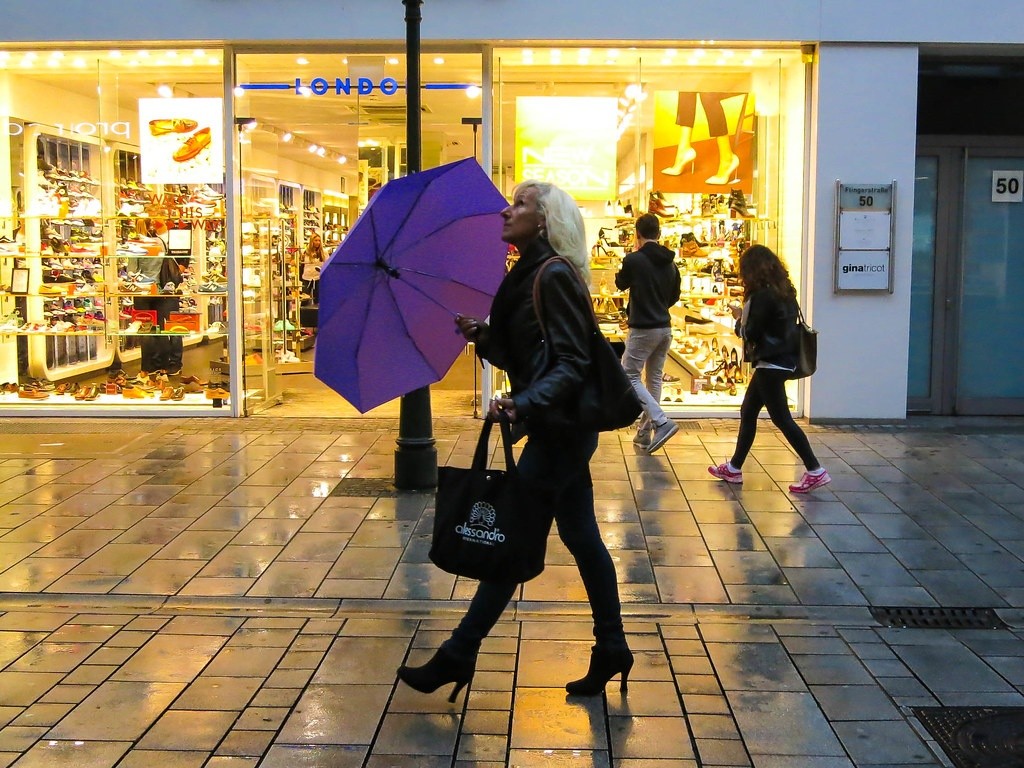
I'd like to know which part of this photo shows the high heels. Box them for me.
[566,648,634,697]
[397,636,477,703]
[705,154,739,185]
[660,149,696,176]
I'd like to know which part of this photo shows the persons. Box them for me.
[397,182,644,703]
[300,233,329,309]
[127,217,183,376]
[709,245,831,493]
[614,213,681,452]
[661,92,748,184]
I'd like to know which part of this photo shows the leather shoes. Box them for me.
[172,127,212,162]
[149,118,197,136]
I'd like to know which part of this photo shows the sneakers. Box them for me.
[647,419,679,453]
[633,430,651,449]
[789,470,831,493]
[708,465,742,484]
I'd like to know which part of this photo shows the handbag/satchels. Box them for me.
[428,410,554,584]
[532,255,646,431]
[789,308,817,385]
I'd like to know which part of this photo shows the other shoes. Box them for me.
[0,163,353,401]
[503,188,755,403]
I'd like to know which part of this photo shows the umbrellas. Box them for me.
[314,157,512,414]
